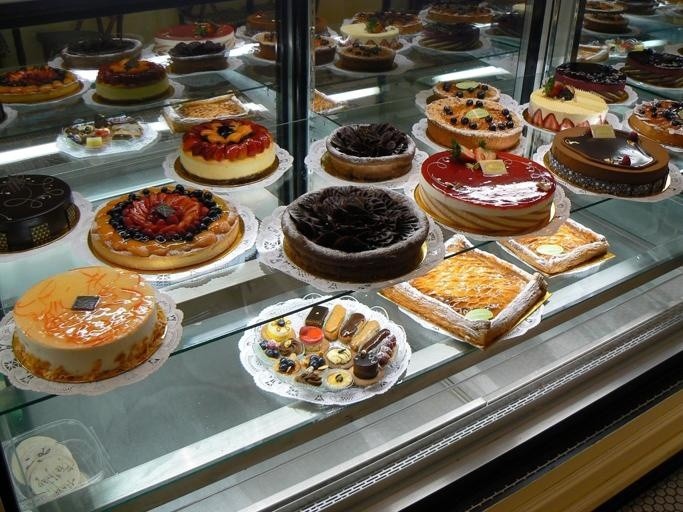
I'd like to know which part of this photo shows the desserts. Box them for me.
[65,114,143,150]
[260,304,398,391]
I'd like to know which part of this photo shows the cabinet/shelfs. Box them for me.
[0,2,683,512]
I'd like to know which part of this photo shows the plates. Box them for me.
[623,98,683,152]
[412,88,520,115]
[8,435,86,498]
[514,101,622,138]
[542,75,638,107]
[494,219,618,283]
[239,292,412,405]
[0,287,185,395]
[159,142,291,189]
[256,198,448,296]
[74,191,256,284]
[1,191,92,266]
[618,57,681,95]
[531,140,683,202]
[410,117,528,157]
[304,133,428,191]
[406,169,573,240]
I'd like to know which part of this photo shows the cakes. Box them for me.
[627,99,682,149]
[281,184,430,272]
[168,40,228,74]
[90,184,239,269]
[1,174,79,251]
[550,125,670,197]
[326,123,417,180]
[524,77,609,133]
[625,50,682,88]
[431,80,501,104]
[236,0,683,91]
[95,59,170,99]
[418,139,557,231]
[499,216,610,274]
[424,96,523,150]
[180,116,275,179]
[554,61,627,103]
[0,65,79,101]
[62,35,143,69]
[154,20,237,54]
[11,266,168,383]
